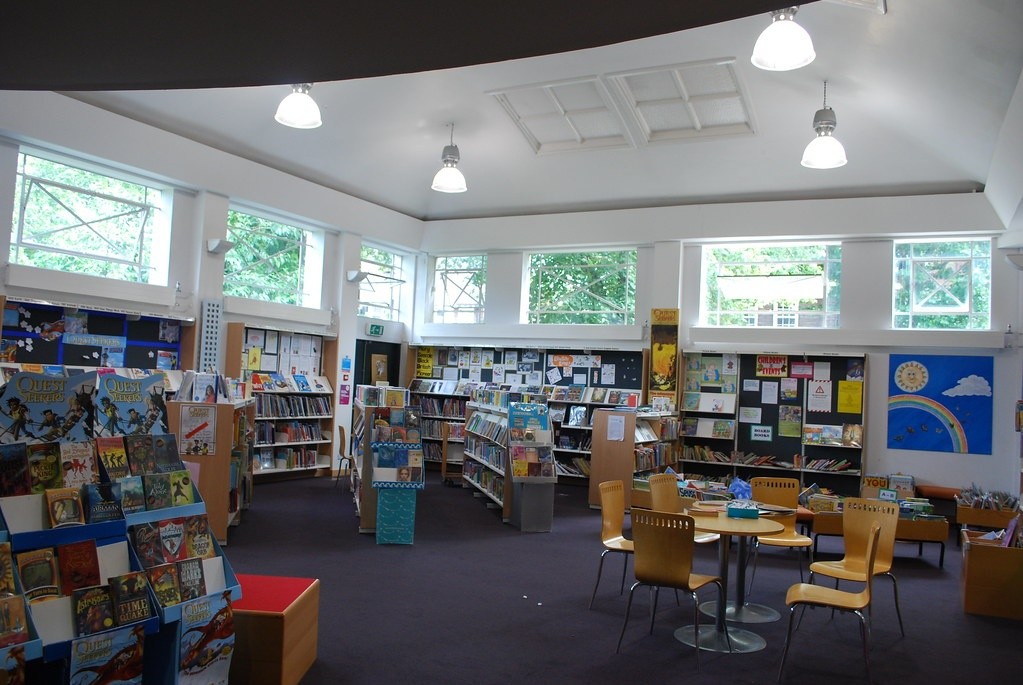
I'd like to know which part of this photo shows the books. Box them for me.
[0,434,227,650]
[233,372,1023,549]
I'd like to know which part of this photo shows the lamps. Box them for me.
[750,5,815,70]
[273,83,323,128]
[431,123,468,193]
[347,270,367,281]
[209,239,233,253]
[801,81,848,167]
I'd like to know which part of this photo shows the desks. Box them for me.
[673,511,785,655]
[228,572,320,685]
[803,508,949,567]
[692,499,792,623]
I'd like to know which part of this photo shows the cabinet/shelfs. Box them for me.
[0,353,868,685]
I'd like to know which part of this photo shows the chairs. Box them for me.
[795,497,906,638]
[588,480,635,611]
[776,520,882,685]
[648,474,720,544]
[616,508,732,668]
[746,477,812,596]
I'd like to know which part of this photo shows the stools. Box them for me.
[916,486,962,545]
